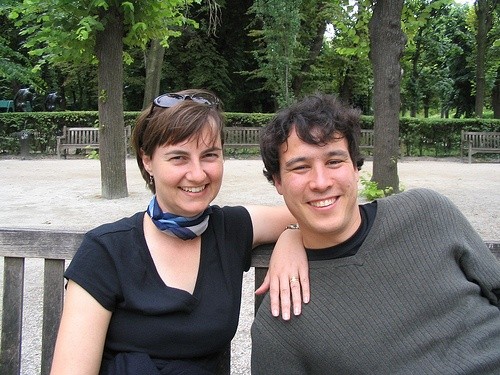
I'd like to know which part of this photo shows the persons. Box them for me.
[48,89,310,375]
[250,93,500,375]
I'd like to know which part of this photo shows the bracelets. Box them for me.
[285,224,299,229]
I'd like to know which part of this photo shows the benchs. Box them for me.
[0,224,500,375]
[460,129,500,164]
[56,125,132,158]
[223,127,261,159]
[359,129,404,164]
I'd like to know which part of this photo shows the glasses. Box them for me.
[149,92,219,115]
